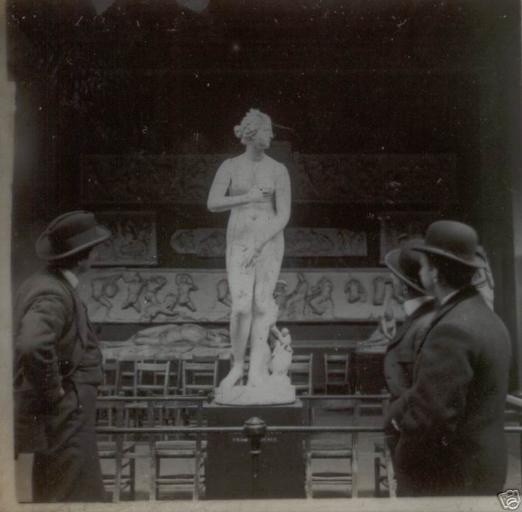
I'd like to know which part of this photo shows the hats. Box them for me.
[36,209,111,261]
[412,219,485,270]
[385,238,431,294]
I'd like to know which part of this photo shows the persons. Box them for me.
[10,210,114,503]
[391,219,515,495]
[207,108,292,391]
[383,239,439,496]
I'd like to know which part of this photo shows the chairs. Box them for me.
[84,351,403,502]
